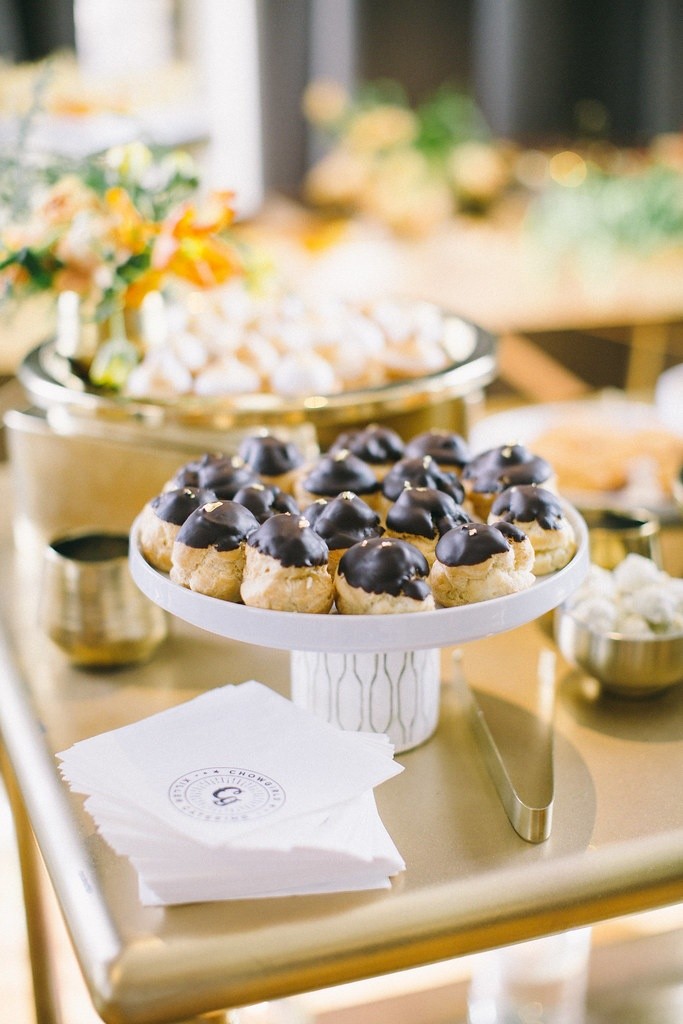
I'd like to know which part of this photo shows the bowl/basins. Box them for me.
[17,306,504,541]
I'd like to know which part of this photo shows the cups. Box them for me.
[467,924,589,1024]
[40,528,167,665]
[554,606,681,700]
[536,500,662,643]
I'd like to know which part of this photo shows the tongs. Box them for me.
[451,644,559,845]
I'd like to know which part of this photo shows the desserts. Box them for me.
[120,280,574,618]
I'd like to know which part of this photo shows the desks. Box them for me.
[0,464,683,1024]
